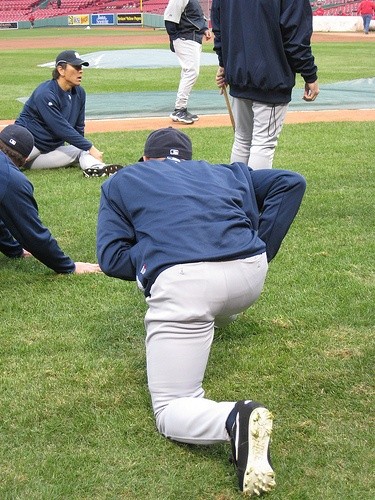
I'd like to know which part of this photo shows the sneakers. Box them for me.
[230,399,276,496]
[170,110,194,124]
[182,109,198,120]
[82,165,124,177]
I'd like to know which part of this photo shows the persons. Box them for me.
[0,124,102,275]
[15,50,124,178]
[57,0,62,8]
[163,0,212,124]
[50,0,54,5]
[96,126,307,495]
[357,0,375,34]
[339,11,347,16]
[210,0,320,170]
[317,4,324,16]
[29,14,35,29]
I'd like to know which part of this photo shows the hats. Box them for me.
[0,124,34,158]
[55,50,89,66]
[138,126,193,162]
